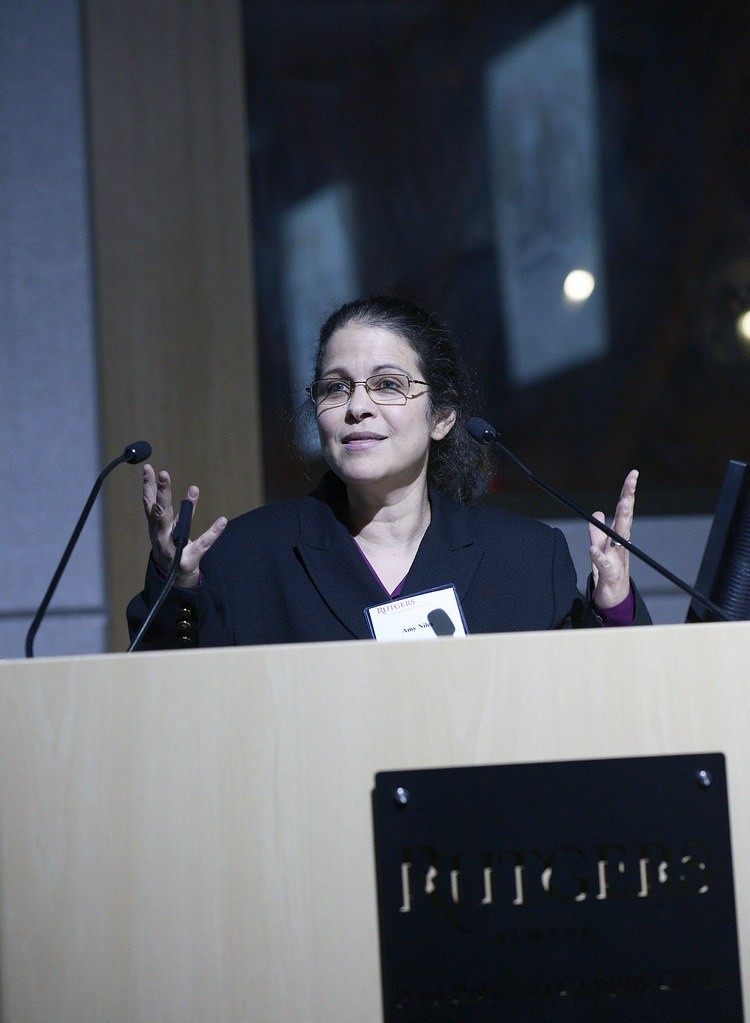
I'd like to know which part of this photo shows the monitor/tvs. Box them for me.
[684,458,750,623]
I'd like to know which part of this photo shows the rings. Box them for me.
[610,539,632,548]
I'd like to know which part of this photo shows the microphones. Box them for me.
[128,500,193,652]
[24,441,153,657]
[467,417,733,624]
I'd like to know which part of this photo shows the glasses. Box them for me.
[306,374,430,405]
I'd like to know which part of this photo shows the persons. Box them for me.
[126,297,654,654]
